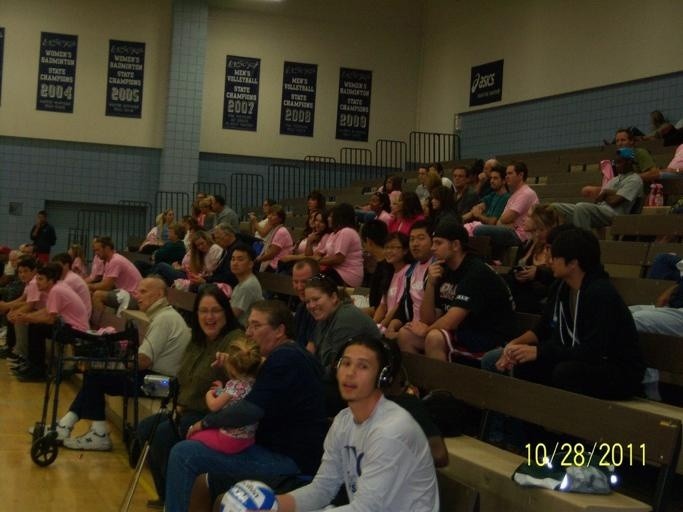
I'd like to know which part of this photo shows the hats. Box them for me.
[431,219,469,243]
[615,147,638,161]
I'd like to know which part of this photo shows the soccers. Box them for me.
[220,480,279,512]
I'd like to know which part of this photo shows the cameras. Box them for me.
[142,374,178,399]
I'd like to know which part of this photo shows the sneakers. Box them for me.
[5,356,47,384]
[61,431,115,454]
[26,422,73,446]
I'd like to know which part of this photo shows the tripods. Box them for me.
[120,401,192,508]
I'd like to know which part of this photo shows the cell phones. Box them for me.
[517,266,524,271]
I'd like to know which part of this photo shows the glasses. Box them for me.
[381,244,403,251]
[244,321,274,332]
[196,306,224,316]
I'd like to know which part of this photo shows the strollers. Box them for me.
[31,314,142,468]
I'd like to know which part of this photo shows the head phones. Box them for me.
[333,335,397,392]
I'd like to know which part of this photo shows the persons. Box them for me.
[388,219,443,341]
[397,213,518,378]
[475,160,502,197]
[186,333,261,453]
[190,222,254,301]
[655,143,683,186]
[627,260,683,337]
[512,200,566,313]
[416,165,431,205]
[356,218,394,324]
[428,163,457,194]
[379,231,411,340]
[361,186,372,205]
[154,231,227,293]
[15,263,89,379]
[303,275,418,395]
[209,194,239,236]
[214,333,441,511]
[390,189,427,234]
[480,228,645,459]
[191,193,214,236]
[26,275,200,451]
[276,207,331,287]
[67,236,105,284]
[544,223,580,297]
[165,301,328,511]
[581,129,660,203]
[7,253,92,371]
[462,165,511,244]
[257,206,294,275]
[377,173,403,200]
[309,203,364,287]
[0,244,40,359]
[181,214,204,254]
[229,243,265,330]
[0,255,49,366]
[69,241,89,279]
[425,186,463,236]
[87,237,144,330]
[0,250,23,287]
[419,172,442,216]
[30,211,56,268]
[308,191,326,213]
[294,258,320,354]
[497,162,540,260]
[133,222,186,279]
[135,284,248,508]
[369,193,393,231]
[643,111,683,145]
[247,199,277,256]
[139,209,177,255]
[451,166,481,215]
[603,126,650,141]
[548,153,643,242]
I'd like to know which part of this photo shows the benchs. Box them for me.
[402,352,683,512]
[439,157,480,168]
[600,241,683,275]
[337,194,380,202]
[442,166,453,178]
[643,138,683,166]
[242,206,265,220]
[512,312,683,377]
[288,212,310,228]
[172,288,197,312]
[128,236,147,253]
[329,188,366,198]
[325,201,342,215]
[545,192,653,215]
[238,221,254,235]
[100,305,176,430]
[611,276,683,308]
[120,251,151,264]
[406,176,419,182]
[486,151,531,166]
[510,161,573,183]
[609,211,682,243]
[531,147,610,174]
[527,180,583,200]
[530,182,600,204]
[278,195,308,213]
[352,178,379,187]
[546,170,610,186]
[262,276,298,305]
[402,181,420,191]
